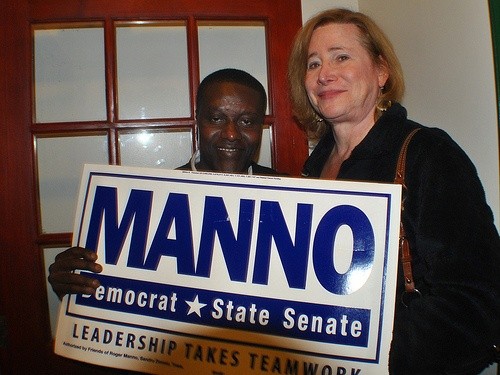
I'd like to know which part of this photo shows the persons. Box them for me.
[48,69,290,300]
[288,8,500,374]
